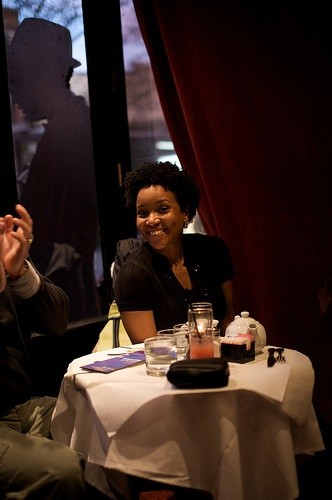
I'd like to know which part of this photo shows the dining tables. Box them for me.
[51,344,324,499]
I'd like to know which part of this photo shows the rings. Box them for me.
[27,235,34,243]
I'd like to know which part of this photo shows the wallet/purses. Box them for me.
[165,358,231,388]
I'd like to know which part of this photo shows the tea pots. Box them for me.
[225,311,266,355]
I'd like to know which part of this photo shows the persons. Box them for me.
[1,203,86,499]
[113,160,237,342]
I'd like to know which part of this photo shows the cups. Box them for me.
[142,302,221,377]
